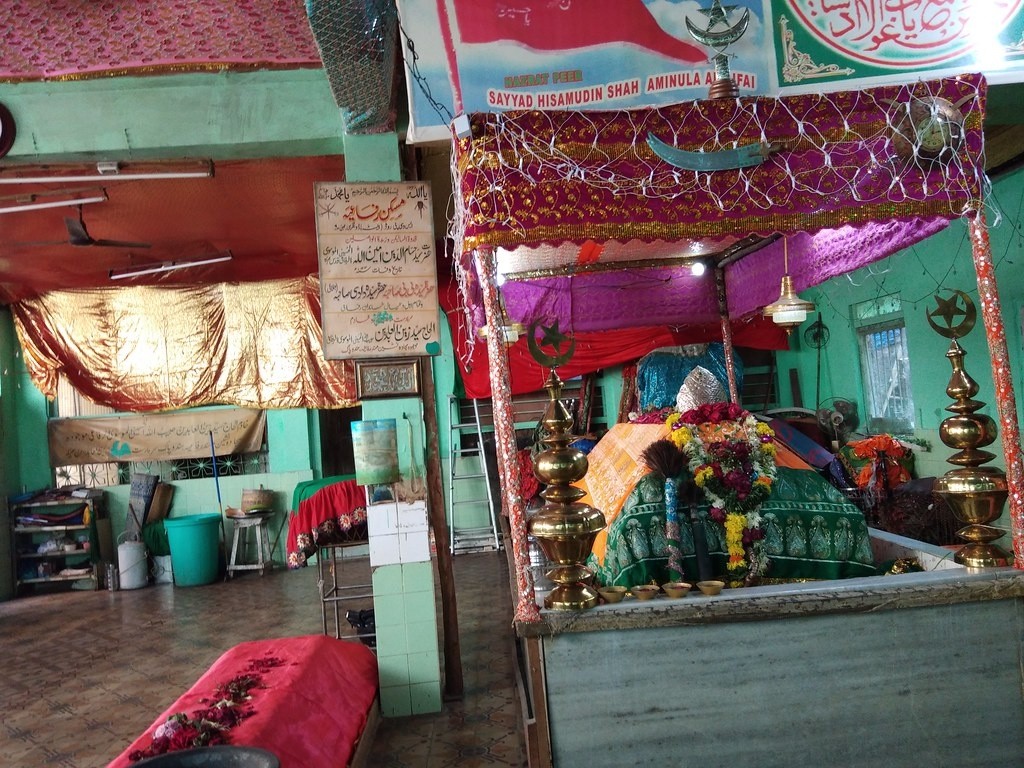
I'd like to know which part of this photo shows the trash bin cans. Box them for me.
[164,512,223,587]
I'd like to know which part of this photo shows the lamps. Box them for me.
[0,157,216,184]
[108,249,234,281]
[763,237,816,336]
[0,187,110,214]
[478,287,528,351]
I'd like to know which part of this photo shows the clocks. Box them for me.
[891,95,967,171]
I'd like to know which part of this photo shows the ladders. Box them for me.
[447,393,501,554]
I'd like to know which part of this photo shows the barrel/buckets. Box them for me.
[117,540,148,589]
[350,418,400,486]
[240,488,273,513]
[162,511,221,587]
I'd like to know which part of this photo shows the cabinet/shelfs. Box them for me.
[6,484,114,599]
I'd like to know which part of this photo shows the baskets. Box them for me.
[240,484,275,514]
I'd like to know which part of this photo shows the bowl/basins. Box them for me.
[64,544,76,551]
[597,586,627,603]
[631,585,660,600]
[662,583,692,597]
[696,581,725,595]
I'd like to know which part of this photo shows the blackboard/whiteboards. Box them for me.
[313,181,442,360]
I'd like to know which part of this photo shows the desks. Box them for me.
[286,474,377,650]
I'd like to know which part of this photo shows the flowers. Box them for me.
[129,697,254,763]
[665,401,780,590]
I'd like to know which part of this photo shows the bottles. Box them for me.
[107,564,118,591]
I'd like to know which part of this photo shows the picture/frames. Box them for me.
[354,357,423,401]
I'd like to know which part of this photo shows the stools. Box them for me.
[225,518,275,578]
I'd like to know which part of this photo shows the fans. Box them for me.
[815,396,856,452]
